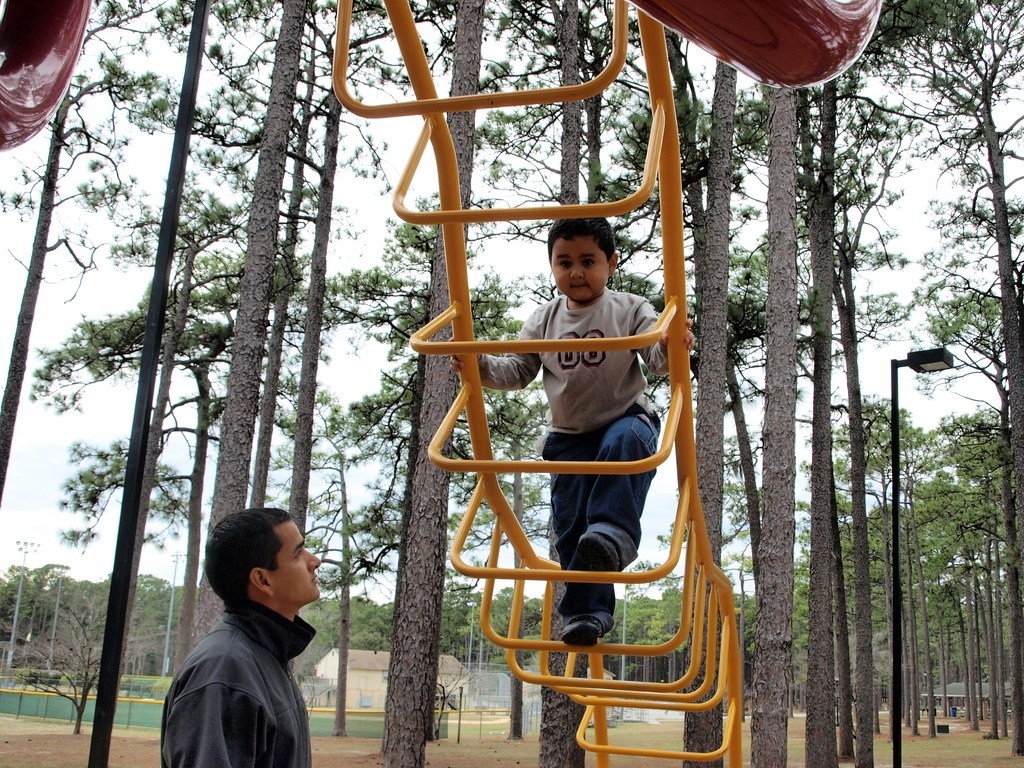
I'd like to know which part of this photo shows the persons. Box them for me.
[444,217,695,646]
[158,507,328,768]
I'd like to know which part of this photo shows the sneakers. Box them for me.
[566,532,621,571]
[559,616,602,645]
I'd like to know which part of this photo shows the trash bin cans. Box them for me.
[949,707,957,717]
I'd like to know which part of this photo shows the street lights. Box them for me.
[890,346,953,768]
[3,540,41,689]
[47,564,71,671]
